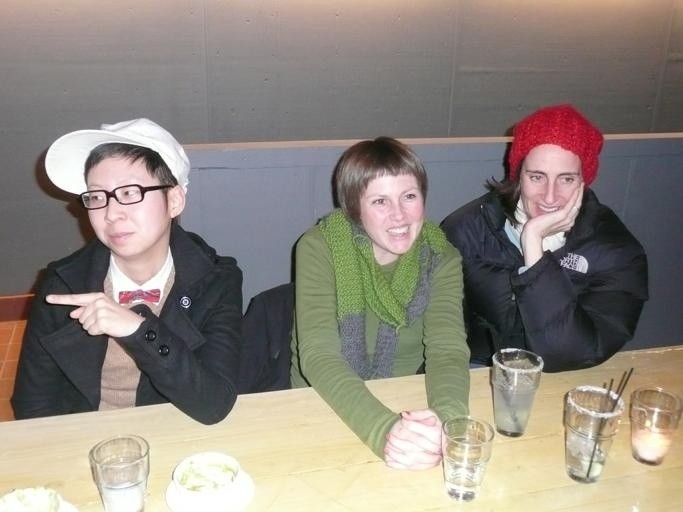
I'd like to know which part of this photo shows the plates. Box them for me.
[164,468,257,512]
[59,494,78,512]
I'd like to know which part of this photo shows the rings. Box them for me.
[572,206,582,212]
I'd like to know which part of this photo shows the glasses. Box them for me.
[81,185,173,209]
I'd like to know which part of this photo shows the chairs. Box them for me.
[0,294,34,423]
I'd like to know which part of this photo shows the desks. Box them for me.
[0,345,683,512]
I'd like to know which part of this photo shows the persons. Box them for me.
[290,136,471,470]
[10,117,244,424]
[439,103,648,374]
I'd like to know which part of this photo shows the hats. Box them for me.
[45,118,190,198]
[507,103,604,189]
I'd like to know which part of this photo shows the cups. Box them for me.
[491,348,545,438]
[441,416,493,502]
[0,486,63,512]
[629,386,683,466]
[90,435,149,512]
[170,452,240,497]
[565,385,625,484]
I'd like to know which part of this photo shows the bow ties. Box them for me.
[119,289,160,302]
